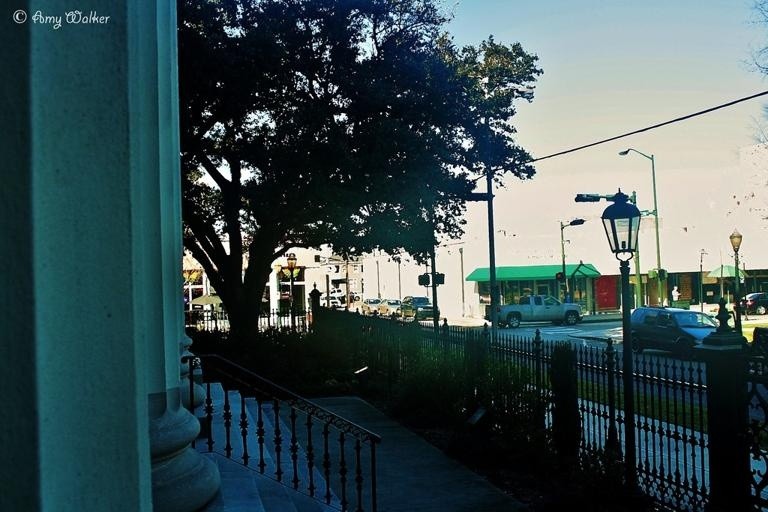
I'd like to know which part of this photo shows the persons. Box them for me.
[672,286,682,301]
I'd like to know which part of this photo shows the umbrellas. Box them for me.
[185,293,223,319]
[706,265,749,311]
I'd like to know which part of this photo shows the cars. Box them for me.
[320,288,440,320]
[733,292,768,315]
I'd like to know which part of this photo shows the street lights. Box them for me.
[601,188,641,496]
[560,148,663,306]
[729,227,743,336]
[287,253,298,333]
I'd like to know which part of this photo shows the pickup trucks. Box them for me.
[484,295,582,328]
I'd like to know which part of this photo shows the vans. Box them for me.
[631,306,720,359]
